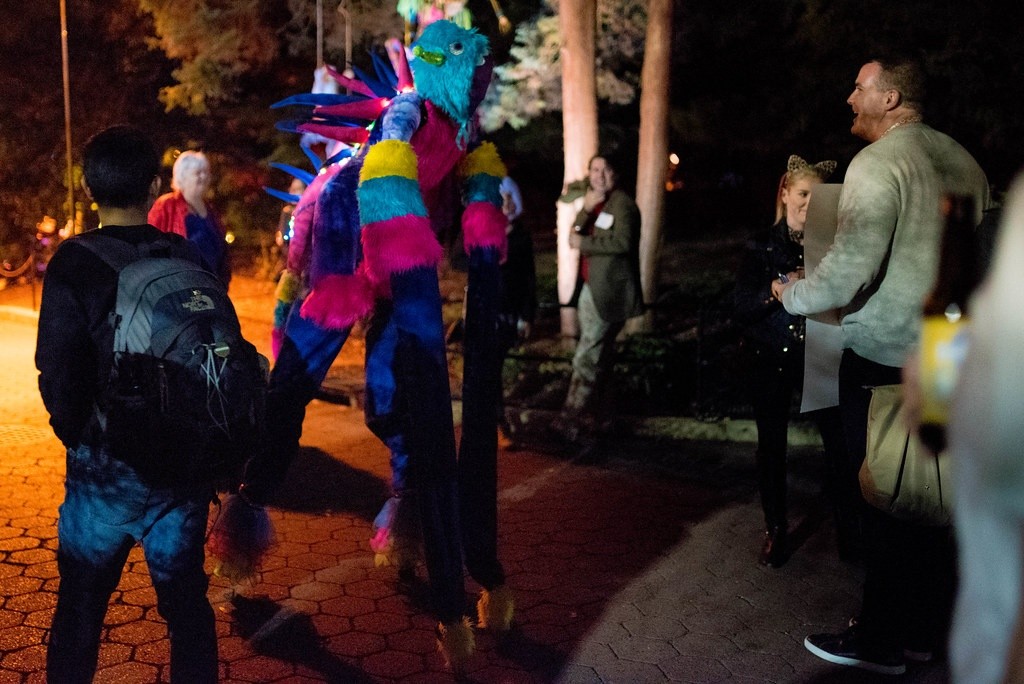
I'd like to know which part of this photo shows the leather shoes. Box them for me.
[760,521,785,566]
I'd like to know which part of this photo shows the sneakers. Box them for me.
[800,620,933,672]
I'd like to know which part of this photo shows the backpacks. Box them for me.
[68,230,271,485]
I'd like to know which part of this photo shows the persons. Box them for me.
[552,155,647,411]
[34,123,218,684]
[749,51,1023,684]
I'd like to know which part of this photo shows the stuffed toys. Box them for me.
[211,20,521,663]
